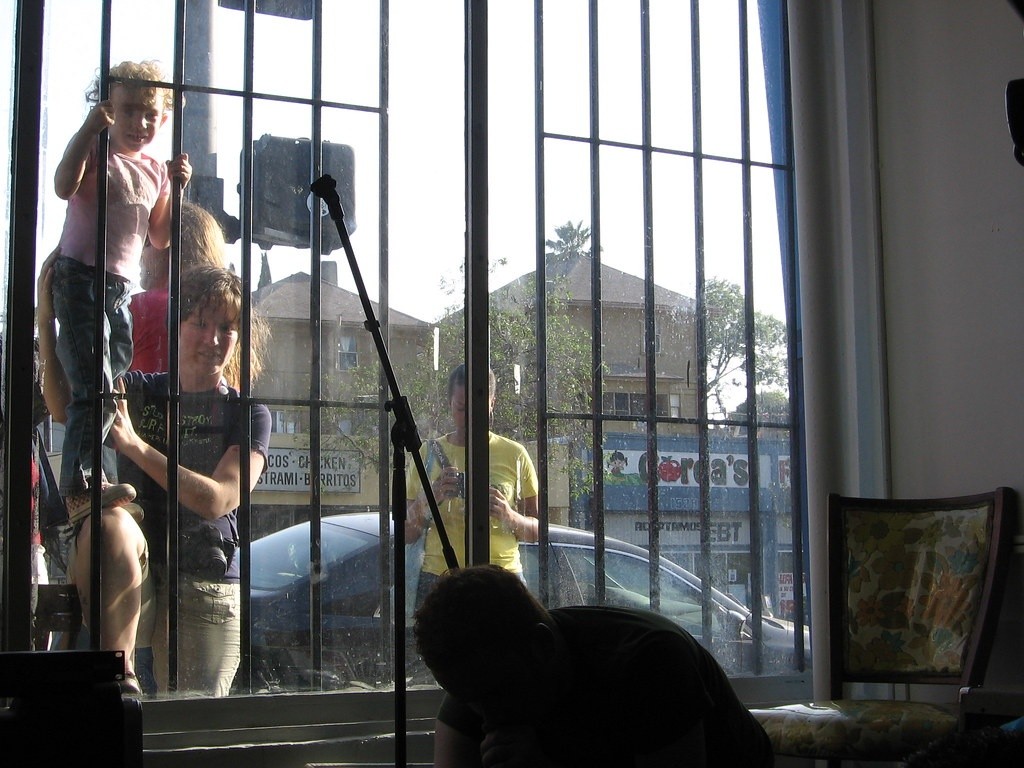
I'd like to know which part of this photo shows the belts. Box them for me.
[149,554,183,571]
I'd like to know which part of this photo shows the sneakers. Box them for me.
[66,480,137,524]
[118,502,144,524]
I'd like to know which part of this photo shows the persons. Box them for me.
[413,564,775,768]
[31,61,272,701]
[404,361,539,616]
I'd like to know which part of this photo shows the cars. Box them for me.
[133,510,812,692]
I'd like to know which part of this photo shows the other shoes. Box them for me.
[120,670,142,697]
